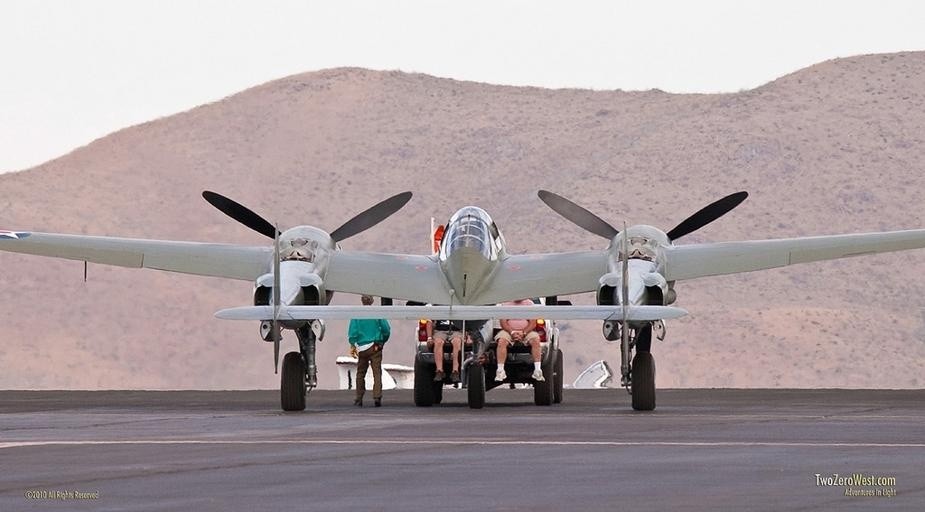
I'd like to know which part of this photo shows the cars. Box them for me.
[406,297,571,407]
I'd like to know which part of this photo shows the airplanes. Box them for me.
[1,190,925,411]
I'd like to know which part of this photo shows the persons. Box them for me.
[426,319,471,384]
[347,294,390,407]
[495,297,547,382]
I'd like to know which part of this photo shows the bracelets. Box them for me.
[522,329,526,336]
[511,331,514,336]
[427,337,432,339]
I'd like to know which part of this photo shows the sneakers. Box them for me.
[495,369,507,382]
[450,371,460,383]
[352,400,361,406]
[433,371,446,382]
[532,369,545,382]
[375,399,381,407]
